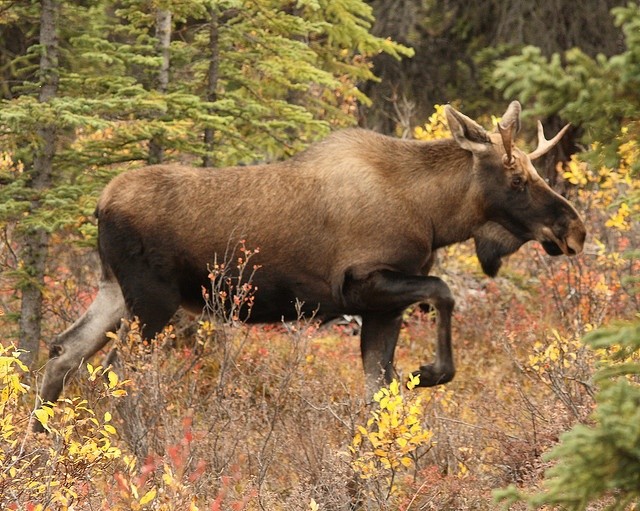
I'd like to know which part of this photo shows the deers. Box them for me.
[17,99,588,459]
